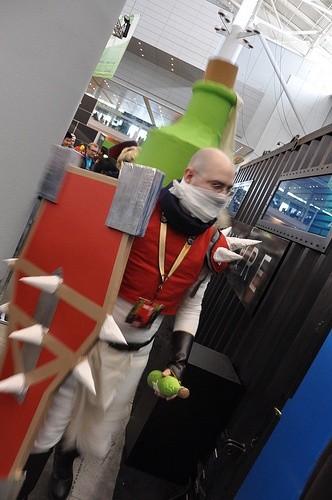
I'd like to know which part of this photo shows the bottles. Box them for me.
[137,79,239,192]
[147,370,189,398]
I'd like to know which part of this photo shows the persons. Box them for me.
[62,132,138,178]
[17,147,263,500]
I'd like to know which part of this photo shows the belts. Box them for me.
[104,335,155,352]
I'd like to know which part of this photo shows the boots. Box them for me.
[49,437,80,499]
[15,446,54,500]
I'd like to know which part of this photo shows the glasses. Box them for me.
[193,168,233,193]
[89,149,97,153]
